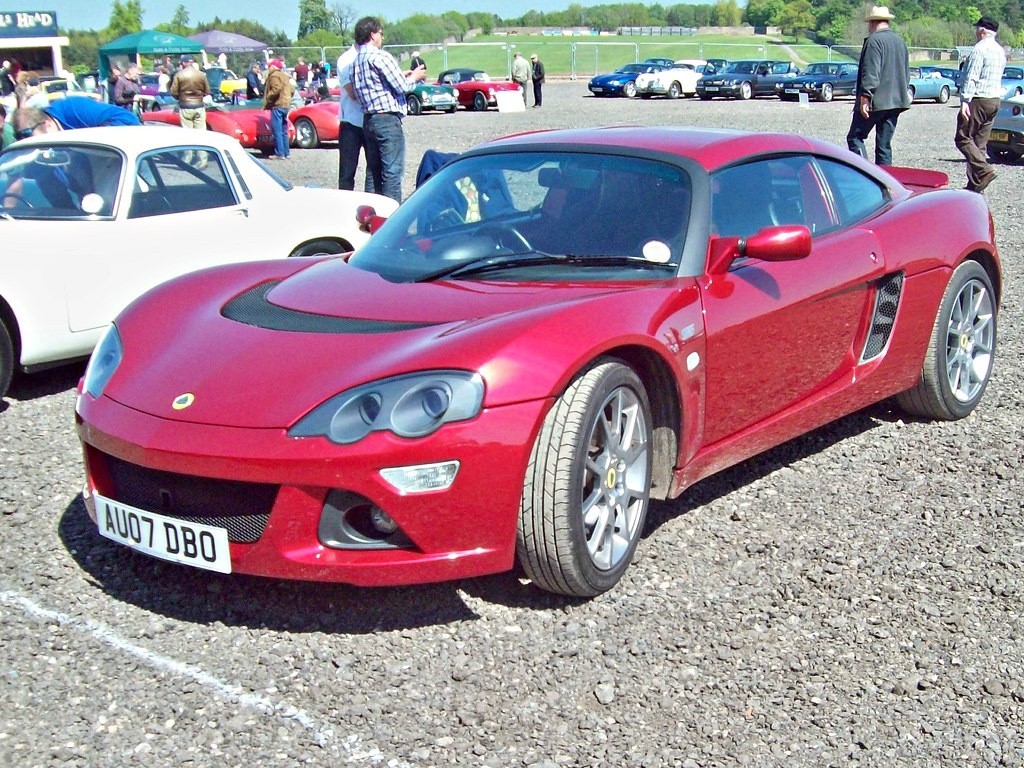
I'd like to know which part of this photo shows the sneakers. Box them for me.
[269,154,284,160]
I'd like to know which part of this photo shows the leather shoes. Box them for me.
[971,169,998,190]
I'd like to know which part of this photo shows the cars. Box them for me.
[906,64,965,104]
[0,120,403,411]
[584,57,860,103]
[986,64,1024,167]
[431,68,527,113]
[404,77,462,116]
[0,65,344,153]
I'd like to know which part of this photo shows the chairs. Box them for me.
[560,160,779,257]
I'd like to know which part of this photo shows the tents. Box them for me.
[97,29,204,107]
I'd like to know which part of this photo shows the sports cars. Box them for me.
[71,123,1007,604]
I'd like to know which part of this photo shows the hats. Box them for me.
[864,6,895,22]
[530,53,538,58]
[513,52,522,57]
[972,17,999,32]
[181,54,192,62]
[267,58,282,68]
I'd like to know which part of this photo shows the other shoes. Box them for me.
[533,103,542,108]
[198,161,209,170]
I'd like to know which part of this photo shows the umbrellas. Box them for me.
[186,30,269,53]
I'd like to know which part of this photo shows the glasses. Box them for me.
[373,30,384,37]
[13,121,45,137]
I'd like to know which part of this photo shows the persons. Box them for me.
[0,104,24,208]
[336,41,412,194]
[847,5,910,166]
[280,56,328,89]
[348,15,426,202]
[153,54,199,75]
[114,62,155,112]
[411,51,426,81]
[13,96,166,214]
[171,54,210,169]
[954,16,1006,191]
[261,59,295,160]
[513,52,545,108]
[246,62,264,98]
[0,57,23,122]
[956,56,966,93]
